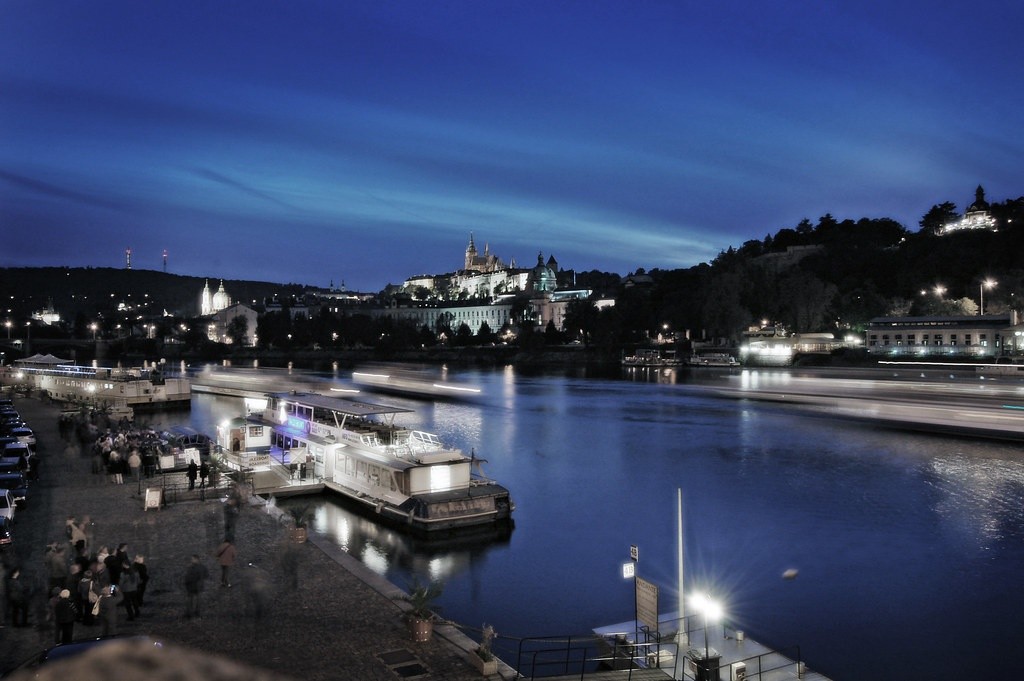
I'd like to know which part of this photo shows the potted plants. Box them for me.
[206,453,224,486]
[389,576,450,641]
[228,467,254,498]
[468,622,499,675]
[277,503,317,544]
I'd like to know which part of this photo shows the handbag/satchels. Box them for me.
[88,581,98,603]
[91,595,102,616]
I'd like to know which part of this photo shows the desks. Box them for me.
[315,414,399,443]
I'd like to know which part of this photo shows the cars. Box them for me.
[0,516,16,552]
[2,443,31,471]
[0,398,14,414]
[0,487,17,521]
[0,412,22,430]
[0,436,16,448]
[0,472,28,507]
[8,427,37,448]
[1,456,31,481]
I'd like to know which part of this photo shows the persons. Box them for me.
[8,512,146,645]
[223,498,237,546]
[232,437,240,452]
[59,411,209,490]
[181,553,212,621]
[18,451,40,482]
[217,534,237,585]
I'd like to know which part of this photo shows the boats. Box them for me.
[190,368,360,400]
[685,351,742,367]
[791,343,1024,377]
[736,323,865,369]
[160,425,211,455]
[352,366,486,399]
[217,388,515,552]
[620,346,686,367]
[0,353,192,413]
